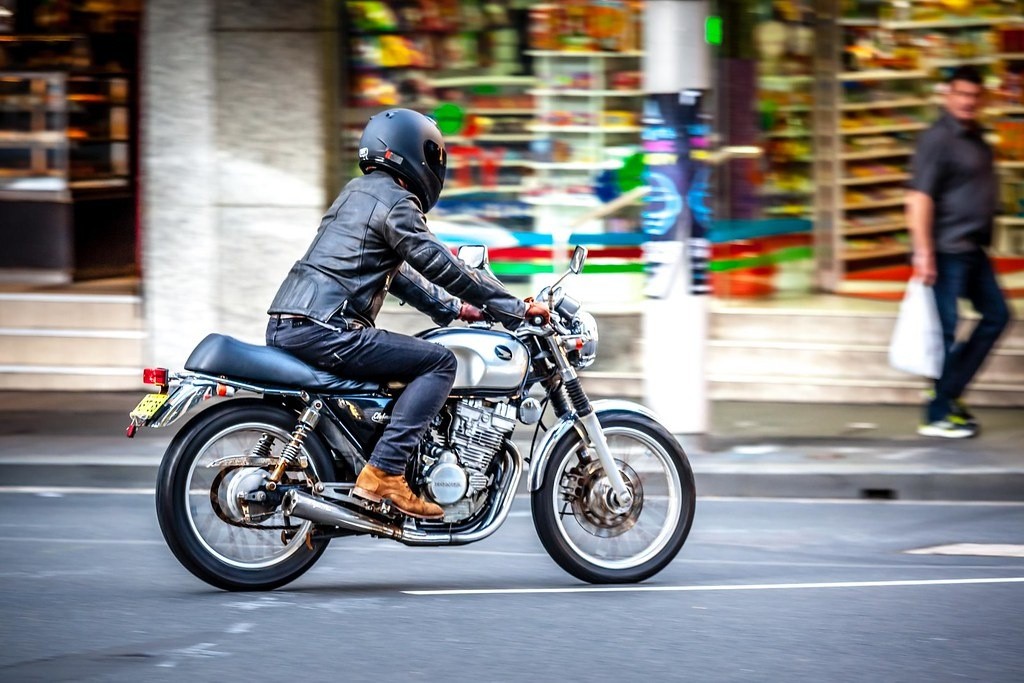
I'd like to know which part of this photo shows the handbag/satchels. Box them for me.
[889,275,945,380]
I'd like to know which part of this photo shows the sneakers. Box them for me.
[352,462,445,519]
[917,407,978,438]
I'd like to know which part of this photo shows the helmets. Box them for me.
[358,108,447,213]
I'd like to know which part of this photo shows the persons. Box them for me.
[265,108,550,520]
[903,65,1012,438]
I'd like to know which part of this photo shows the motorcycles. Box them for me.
[125,242,698,593]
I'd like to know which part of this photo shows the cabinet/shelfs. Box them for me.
[0,32,140,271]
[337,0,1024,263]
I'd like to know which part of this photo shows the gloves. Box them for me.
[523,301,550,327]
[455,303,484,324]
